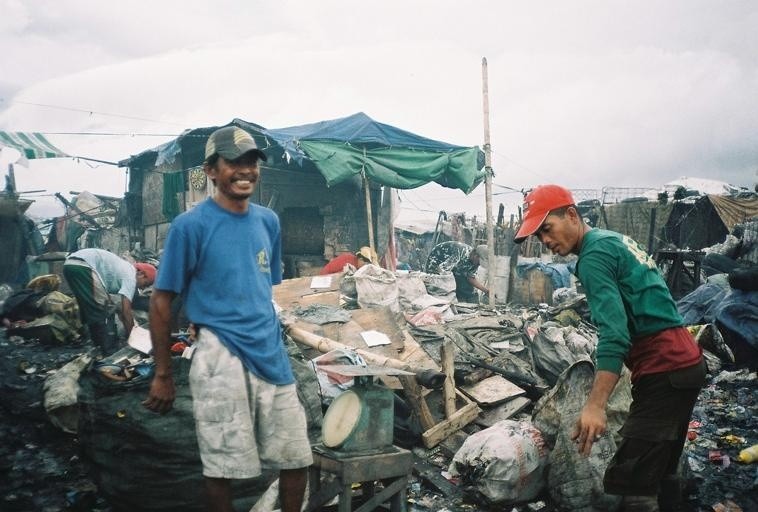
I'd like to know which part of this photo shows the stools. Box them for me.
[309,438,411,511]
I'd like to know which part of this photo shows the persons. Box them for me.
[514,184,708,512]
[64,248,156,355]
[426,241,496,300]
[140,125,314,512]
[320,246,377,275]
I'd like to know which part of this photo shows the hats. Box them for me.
[204,126,267,161]
[514,185,575,243]
[134,262,157,285]
[476,245,489,261]
[356,246,372,262]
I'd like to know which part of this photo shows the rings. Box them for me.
[596,435,601,440]
